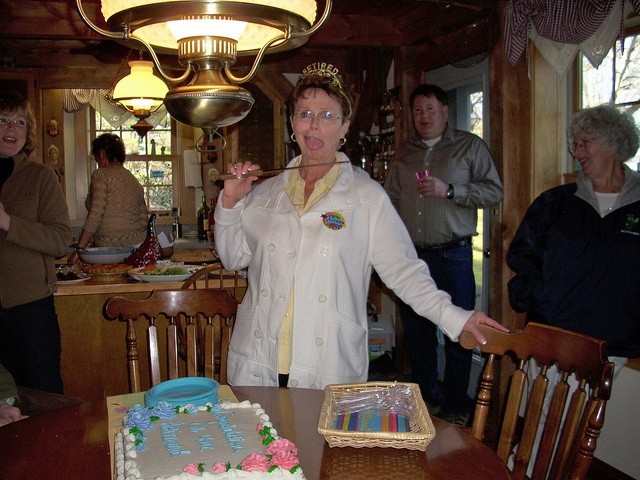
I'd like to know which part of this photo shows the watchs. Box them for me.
[447,184,455,199]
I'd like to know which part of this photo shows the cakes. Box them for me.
[113,400,306,480]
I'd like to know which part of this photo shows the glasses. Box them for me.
[568,134,602,150]
[0,116,27,127]
[294,109,345,123]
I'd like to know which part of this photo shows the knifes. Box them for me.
[210,161,350,183]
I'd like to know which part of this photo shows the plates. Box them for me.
[127,266,205,282]
[55,274,91,285]
[144,377,221,406]
[82,268,129,276]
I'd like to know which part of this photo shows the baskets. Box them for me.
[317,381,436,452]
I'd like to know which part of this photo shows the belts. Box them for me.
[417,234,477,254]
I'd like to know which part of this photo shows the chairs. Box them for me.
[102,289,239,394]
[458,321,614,480]
[180,264,247,376]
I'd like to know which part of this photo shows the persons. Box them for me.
[506,101,639,361]
[0,91,73,397]
[382,84,505,422]
[211,61,511,390]
[65,133,149,266]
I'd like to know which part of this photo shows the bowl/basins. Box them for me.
[78,246,133,264]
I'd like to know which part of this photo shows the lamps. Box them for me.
[75,1,333,153]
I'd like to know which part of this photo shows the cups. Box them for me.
[415,169,430,184]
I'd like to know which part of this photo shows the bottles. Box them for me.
[208,194,216,242]
[173,216,183,239]
[196,195,210,240]
[359,144,395,180]
